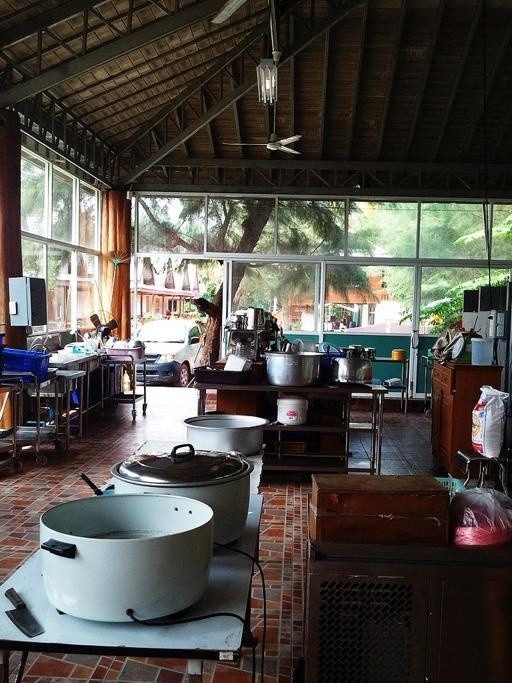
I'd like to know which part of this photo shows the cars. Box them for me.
[131,318,206,387]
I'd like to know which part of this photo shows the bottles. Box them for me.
[246,307,255,330]
[471,337,494,366]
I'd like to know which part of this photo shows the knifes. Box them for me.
[4,587,45,638]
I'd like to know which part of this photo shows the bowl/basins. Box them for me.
[110,340,144,349]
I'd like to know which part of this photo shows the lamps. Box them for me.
[255,20,279,105]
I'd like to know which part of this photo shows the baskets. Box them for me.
[436,477,468,503]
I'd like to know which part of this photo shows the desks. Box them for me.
[0,492,264,683]
[370,356,409,416]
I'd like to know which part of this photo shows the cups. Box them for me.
[291,339,317,352]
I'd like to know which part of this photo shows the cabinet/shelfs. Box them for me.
[431,356,504,477]
[1,347,148,472]
[196,380,388,476]
[303,538,512,682]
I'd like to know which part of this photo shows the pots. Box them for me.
[281,342,299,352]
[183,414,270,457]
[39,491,215,625]
[266,351,327,386]
[340,344,376,359]
[111,443,255,548]
[329,357,372,384]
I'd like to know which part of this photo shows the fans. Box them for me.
[222,99,302,156]
[81,310,120,349]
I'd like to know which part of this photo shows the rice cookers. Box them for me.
[276,395,309,426]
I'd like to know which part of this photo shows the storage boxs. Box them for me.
[308,473,451,549]
